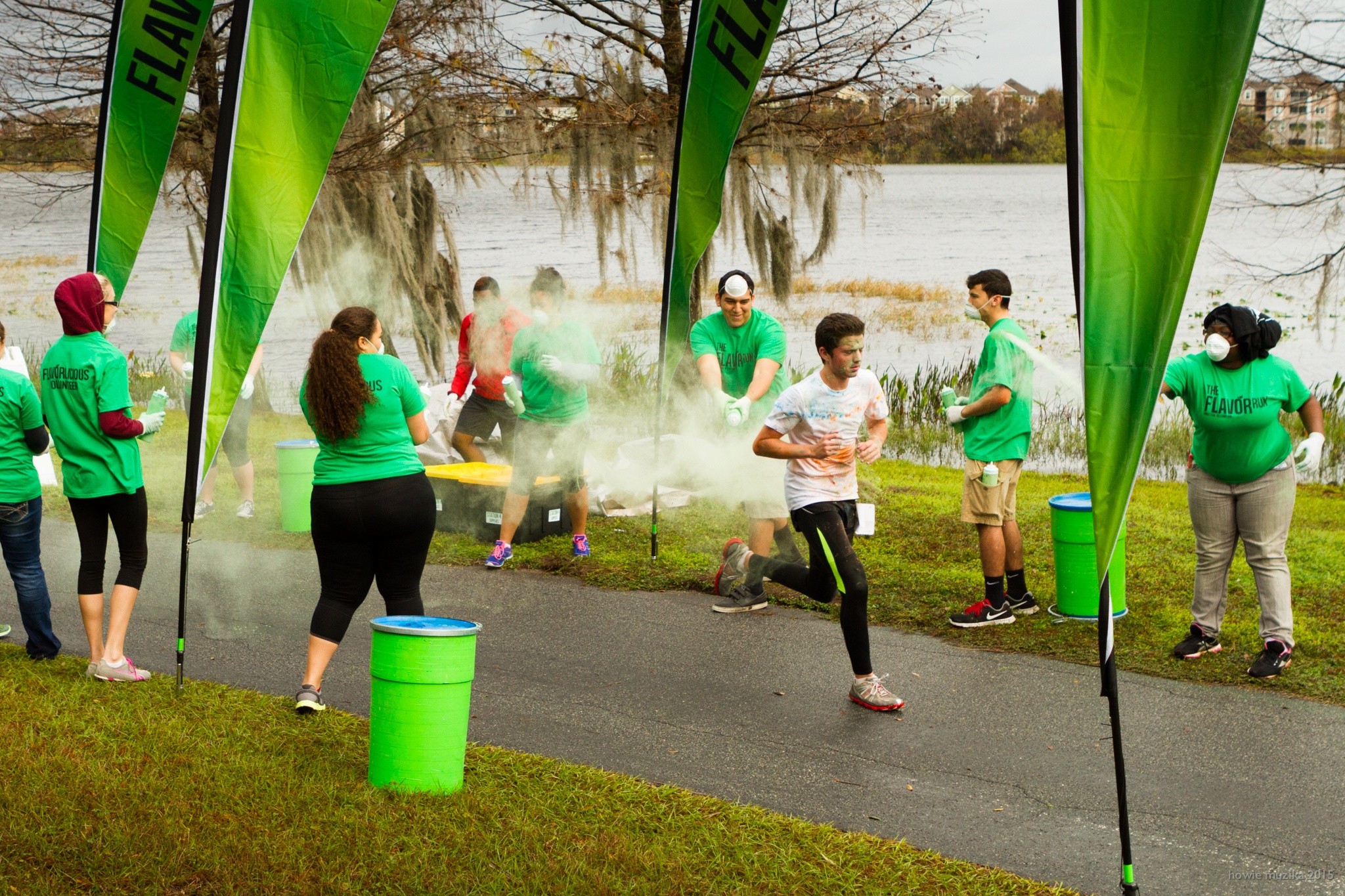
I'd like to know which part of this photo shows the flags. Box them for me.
[181,0,400,523]
[659,0,790,411]
[87,0,222,304]
[1059,1,1266,695]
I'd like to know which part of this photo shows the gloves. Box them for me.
[240,375,255,399]
[945,405,968,424]
[443,393,458,418]
[711,391,738,418]
[1294,431,1326,475]
[730,396,752,425]
[181,370,193,383]
[540,354,564,372]
[940,396,970,411]
[137,412,166,436]
[502,389,522,408]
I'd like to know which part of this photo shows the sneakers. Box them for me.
[194,500,215,520]
[763,556,810,581]
[848,674,905,710]
[712,584,768,612]
[714,536,749,597]
[485,540,513,567]
[573,534,591,557]
[1003,592,1040,614]
[948,598,1015,627]
[1173,625,1222,659]
[236,500,256,519]
[94,658,151,681]
[85,662,98,679]
[1247,639,1292,678]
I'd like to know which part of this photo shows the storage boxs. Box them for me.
[423,462,572,546]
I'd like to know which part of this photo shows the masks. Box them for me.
[365,337,385,355]
[718,274,753,297]
[1205,333,1238,362]
[964,295,1010,321]
[103,318,116,335]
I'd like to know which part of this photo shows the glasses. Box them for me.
[1203,329,1233,339]
[104,300,120,308]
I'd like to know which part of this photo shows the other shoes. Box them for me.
[0,625,12,638]
[296,684,326,710]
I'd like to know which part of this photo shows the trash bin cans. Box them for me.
[1047,494,1128,619]
[276,441,321,534]
[366,617,480,793]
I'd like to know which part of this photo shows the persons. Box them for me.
[290,304,440,711]
[37,273,164,682]
[0,322,63,660]
[1159,303,1325,678]
[169,309,263,518]
[443,265,601,565]
[752,313,906,712]
[944,270,1040,627]
[688,270,810,613]
